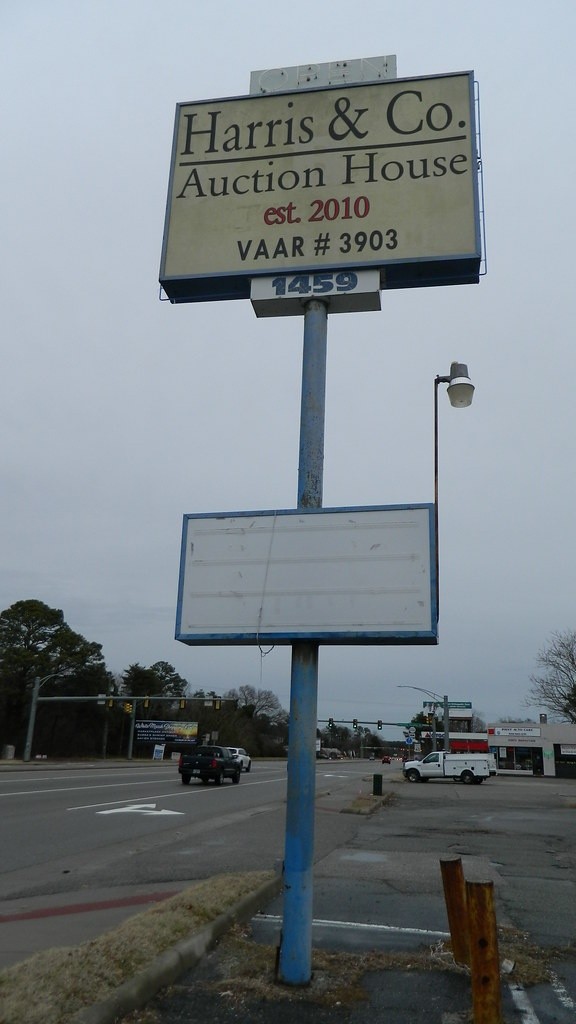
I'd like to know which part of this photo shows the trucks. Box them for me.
[401,751,497,786]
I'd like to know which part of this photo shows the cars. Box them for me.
[177,747,243,789]
[382,757,391,764]
[369,756,375,761]
[227,748,252,773]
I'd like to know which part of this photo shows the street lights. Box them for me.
[397,685,450,753]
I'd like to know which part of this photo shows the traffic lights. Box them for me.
[126,703,133,713]
[328,718,333,728]
[353,718,358,729]
[378,719,382,730]
